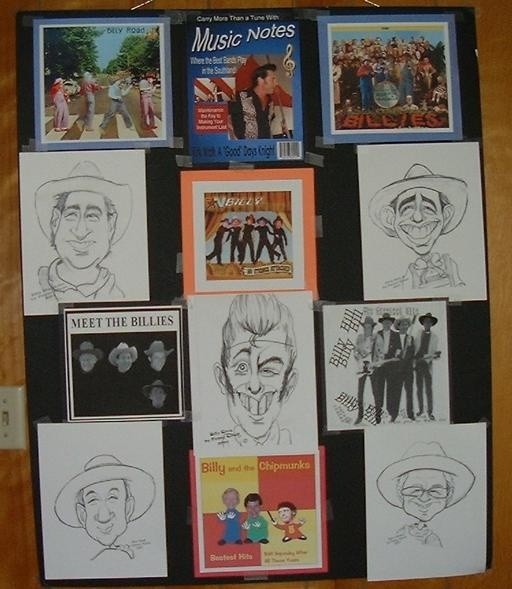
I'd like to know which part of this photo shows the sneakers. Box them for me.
[54,120,157,132]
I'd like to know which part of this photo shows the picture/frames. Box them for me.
[190,446,330,577]
[32,16,173,150]
[315,15,463,144]
[179,167,319,304]
[59,301,192,425]
[315,297,453,438]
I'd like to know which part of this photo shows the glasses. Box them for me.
[397,485,449,499]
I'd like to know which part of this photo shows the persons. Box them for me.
[244,493,262,518]
[277,501,296,523]
[222,487,240,509]
[206,213,288,264]
[194,80,236,103]
[51,70,161,132]
[354,313,441,425]
[332,36,448,113]
[72,340,179,417]
[227,63,289,140]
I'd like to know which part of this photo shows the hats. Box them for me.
[359,312,437,327]
[143,380,173,398]
[72,340,102,361]
[34,161,133,245]
[369,163,469,236]
[220,214,284,225]
[109,342,138,366]
[377,442,475,509]
[54,455,156,527]
[144,340,174,358]
